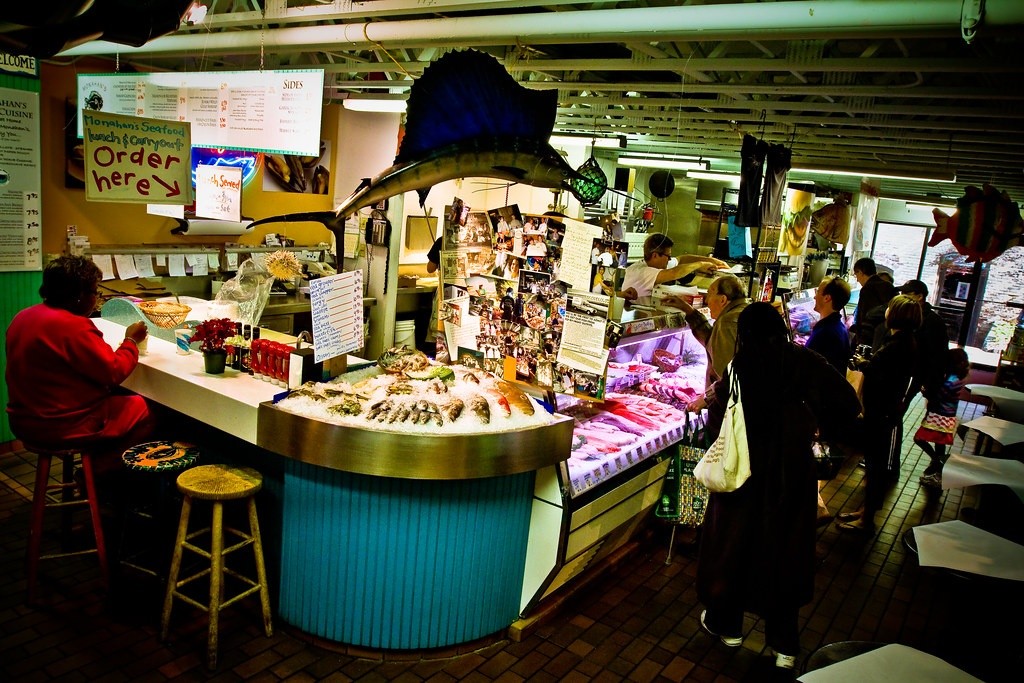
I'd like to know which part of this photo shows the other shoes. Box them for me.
[923,460,941,477]
[772,648,795,670]
[700,609,743,647]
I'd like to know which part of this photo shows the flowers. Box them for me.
[189,318,238,355]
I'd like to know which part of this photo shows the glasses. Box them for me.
[662,253,671,260]
[92,290,103,299]
[853,273,862,278]
[815,289,823,295]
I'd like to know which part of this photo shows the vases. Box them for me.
[204,350,227,374]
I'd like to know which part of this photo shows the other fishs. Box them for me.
[364,377,536,429]
[403,364,456,383]
[451,365,495,385]
[926,182,1024,262]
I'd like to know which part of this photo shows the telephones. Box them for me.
[278,281,296,294]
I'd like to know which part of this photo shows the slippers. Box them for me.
[837,512,857,520]
[835,522,876,532]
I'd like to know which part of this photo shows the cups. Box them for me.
[175,328,193,356]
[136,336,148,356]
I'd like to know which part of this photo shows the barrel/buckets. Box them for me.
[395,320,415,349]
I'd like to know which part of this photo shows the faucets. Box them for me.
[296,330,313,349]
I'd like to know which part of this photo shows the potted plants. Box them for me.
[806,252,829,285]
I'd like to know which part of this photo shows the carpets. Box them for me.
[958,368,997,405]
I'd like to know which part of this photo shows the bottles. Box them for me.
[750,272,760,301]
[225,322,295,389]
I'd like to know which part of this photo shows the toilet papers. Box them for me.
[181,218,255,236]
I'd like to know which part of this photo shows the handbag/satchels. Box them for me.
[654,407,710,529]
[692,360,752,494]
[846,366,865,418]
[812,441,845,481]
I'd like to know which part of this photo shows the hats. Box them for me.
[895,279,929,296]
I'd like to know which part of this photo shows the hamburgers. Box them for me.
[784,190,813,254]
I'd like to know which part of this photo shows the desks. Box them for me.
[956,415,1024,457]
[964,384,1024,414]
[902,519,1024,582]
[796,641,985,683]
[942,455,1024,503]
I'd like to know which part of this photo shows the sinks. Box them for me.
[287,341,369,365]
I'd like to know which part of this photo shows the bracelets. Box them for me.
[123,337,137,347]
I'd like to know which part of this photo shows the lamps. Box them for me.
[548,133,627,148]
[570,117,608,207]
[787,162,957,184]
[343,93,410,113]
[617,155,710,171]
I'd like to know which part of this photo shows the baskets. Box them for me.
[653,349,679,373]
[139,300,191,327]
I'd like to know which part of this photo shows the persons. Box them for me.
[426,236,443,274]
[834,294,923,534]
[692,302,861,669]
[4,254,156,555]
[441,199,628,398]
[660,276,751,450]
[620,231,730,298]
[803,275,851,529]
[848,258,969,489]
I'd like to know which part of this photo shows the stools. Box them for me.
[23,445,112,591]
[119,439,272,671]
